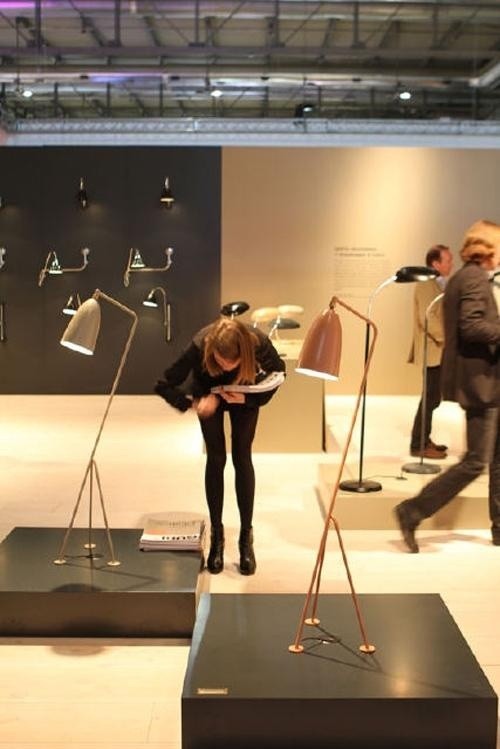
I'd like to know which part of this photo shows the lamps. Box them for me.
[402,291,446,474]
[54,288,139,566]
[220,301,303,356]
[287,295,379,654]
[37,175,176,342]
[337,265,440,493]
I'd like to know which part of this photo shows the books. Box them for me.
[136,515,208,554]
[208,370,287,396]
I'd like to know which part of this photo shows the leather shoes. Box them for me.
[393,498,423,553]
[410,438,448,459]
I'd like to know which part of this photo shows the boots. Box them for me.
[207,523,224,574]
[239,526,256,576]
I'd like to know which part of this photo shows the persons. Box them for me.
[392,216,500,557]
[404,242,455,460]
[148,317,288,577]
